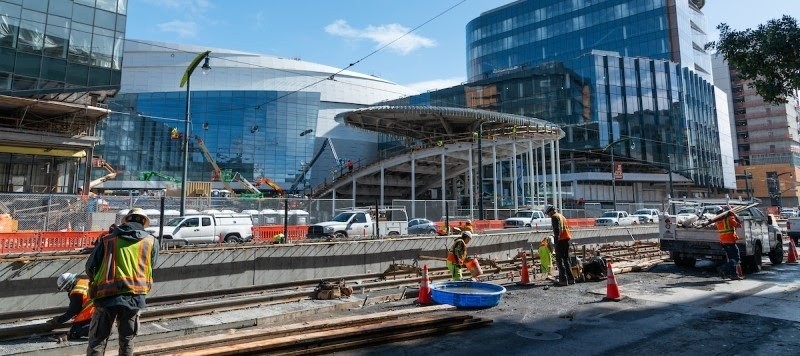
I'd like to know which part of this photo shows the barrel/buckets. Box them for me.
[465,258,483,277]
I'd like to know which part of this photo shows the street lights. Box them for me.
[177,49,213,217]
[772,169,793,194]
[476,119,496,220]
[602,136,636,211]
[664,166,702,198]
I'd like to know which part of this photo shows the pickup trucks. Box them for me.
[505,210,554,230]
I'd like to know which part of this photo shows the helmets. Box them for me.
[121,208,151,228]
[466,220,471,223]
[543,204,555,214]
[463,231,472,239]
[56,272,77,292]
[714,206,722,213]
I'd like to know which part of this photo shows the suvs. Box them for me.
[593,211,632,227]
[629,209,661,223]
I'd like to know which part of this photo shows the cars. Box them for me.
[676,205,737,216]
[779,209,800,246]
[408,217,437,235]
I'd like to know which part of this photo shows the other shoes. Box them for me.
[557,279,576,286]
[730,274,744,280]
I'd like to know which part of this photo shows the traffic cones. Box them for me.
[412,264,436,306]
[785,242,800,265]
[790,239,800,260]
[602,262,625,302]
[515,253,537,287]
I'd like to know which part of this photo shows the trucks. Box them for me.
[151,213,254,248]
[655,205,784,276]
[306,208,409,240]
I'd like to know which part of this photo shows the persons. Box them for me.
[446,231,471,281]
[85,207,160,356]
[544,205,575,286]
[716,206,744,279]
[348,160,353,171]
[538,234,554,273]
[458,219,473,234]
[44,273,95,338]
[76,188,83,195]
